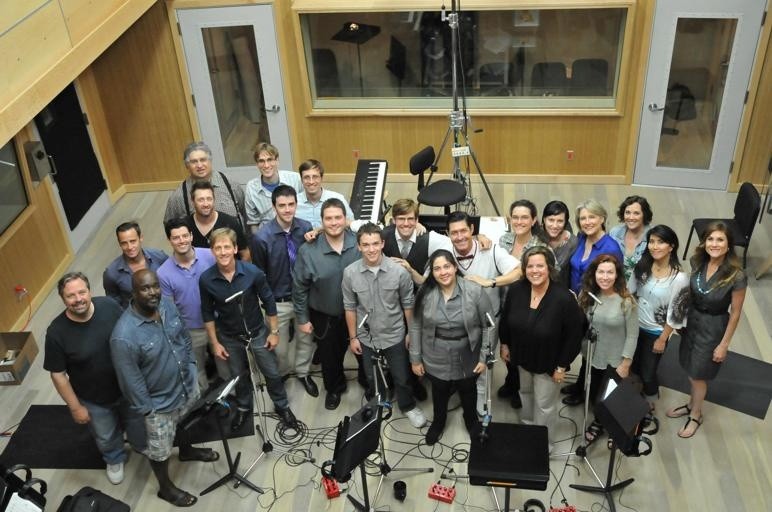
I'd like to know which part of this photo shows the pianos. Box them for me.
[350,160,388,226]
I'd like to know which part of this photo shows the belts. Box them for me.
[222,325,264,341]
[434,331,468,341]
[400,240,409,259]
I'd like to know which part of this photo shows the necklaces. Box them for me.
[697,266,731,294]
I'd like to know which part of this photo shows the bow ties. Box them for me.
[456,255,474,262]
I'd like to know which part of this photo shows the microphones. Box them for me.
[588,290,603,305]
[485,310,495,328]
[226,290,245,302]
[359,308,372,330]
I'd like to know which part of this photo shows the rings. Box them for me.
[558,380,561,383]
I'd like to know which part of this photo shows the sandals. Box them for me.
[608,433,615,448]
[678,412,703,438]
[585,419,602,440]
[666,403,692,417]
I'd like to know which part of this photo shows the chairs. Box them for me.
[530,62,567,97]
[570,58,608,98]
[408,147,467,215]
[682,182,761,270]
[479,61,514,98]
[311,47,341,98]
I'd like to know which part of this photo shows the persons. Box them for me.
[178,181,253,381]
[578,254,638,450]
[294,159,354,365]
[293,198,389,411]
[559,200,624,405]
[156,219,240,407]
[244,142,304,340]
[42,271,150,485]
[198,227,297,431]
[250,184,319,398]
[538,200,578,287]
[103,221,169,306]
[390,211,521,421]
[499,246,583,455]
[409,250,497,446]
[340,219,426,428]
[497,199,560,408]
[164,142,246,241]
[108,270,219,508]
[626,226,690,430]
[304,199,492,403]
[665,221,748,437]
[606,195,652,298]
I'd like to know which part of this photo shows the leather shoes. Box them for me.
[510,392,524,409]
[276,404,297,427]
[326,391,342,410]
[425,421,441,445]
[231,407,251,431]
[561,383,576,393]
[467,424,481,440]
[498,383,510,398]
[562,396,584,405]
[302,375,319,397]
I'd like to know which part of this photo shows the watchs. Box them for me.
[270,329,279,335]
[554,368,565,373]
[490,277,496,288]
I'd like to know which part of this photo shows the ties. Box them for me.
[286,232,298,280]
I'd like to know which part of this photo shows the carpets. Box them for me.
[0,404,110,469]
[654,332,772,420]
[173,382,255,446]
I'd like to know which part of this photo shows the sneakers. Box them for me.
[106,462,124,484]
[132,445,150,456]
[404,405,427,428]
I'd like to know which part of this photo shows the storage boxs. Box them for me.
[0,328,40,385]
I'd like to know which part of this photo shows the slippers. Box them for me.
[157,487,197,507]
[180,447,219,462]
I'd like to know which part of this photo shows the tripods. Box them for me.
[359,332,434,511]
[200,409,265,496]
[548,304,609,488]
[441,329,502,512]
[412,0,502,215]
[570,438,635,512]
[234,304,315,490]
